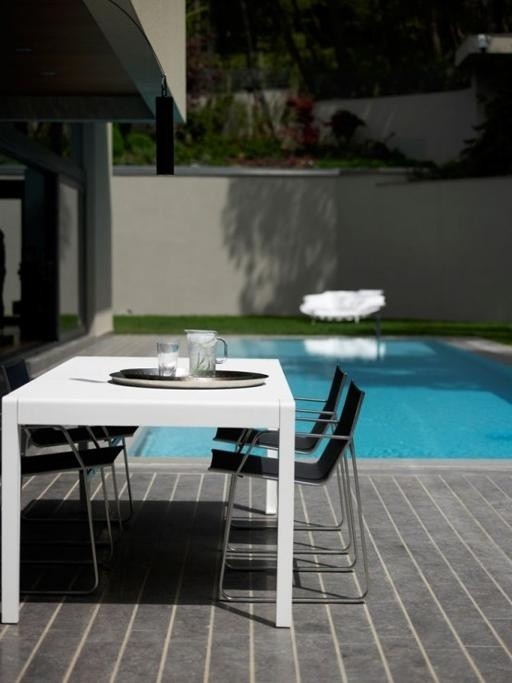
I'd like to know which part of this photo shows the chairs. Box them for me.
[213,363,352,557]
[208,381,371,606]
[4,358,139,546]
[0,383,124,594]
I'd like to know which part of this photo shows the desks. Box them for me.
[0,355,297,628]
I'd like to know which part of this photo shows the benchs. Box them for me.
[300,288,385,337]
[305,337,387,366]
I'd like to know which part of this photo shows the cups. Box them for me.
[156,341,179,380]
[184,328,228,379]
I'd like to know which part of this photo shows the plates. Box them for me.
[110,369,270,387]
[120,369,165,378]
